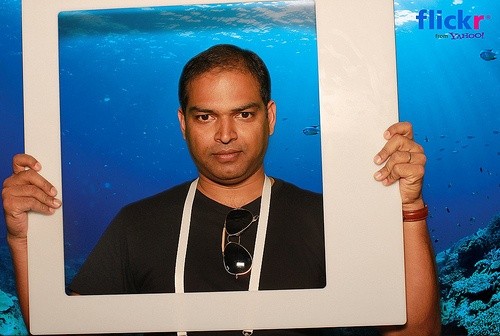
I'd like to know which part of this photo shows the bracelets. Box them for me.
[402,205,429,222]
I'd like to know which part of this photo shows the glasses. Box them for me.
[220,207,258,278]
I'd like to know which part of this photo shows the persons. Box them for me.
[2,43,442,336]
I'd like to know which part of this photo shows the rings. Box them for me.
[406,151,412,163]
